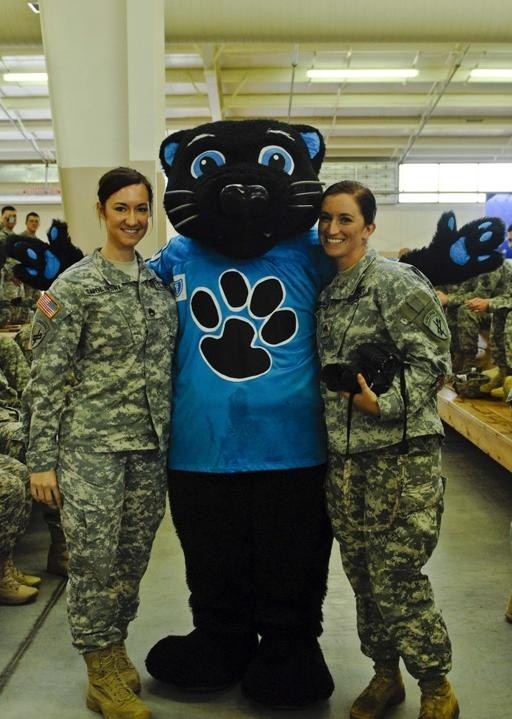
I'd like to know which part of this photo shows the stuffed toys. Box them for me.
[9,120,506,709]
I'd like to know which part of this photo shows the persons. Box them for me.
[27,168,179,719]
[399,224,512,407]
[315,180,460,719]
[1,205,69,606]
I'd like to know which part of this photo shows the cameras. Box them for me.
[321,342,397,396]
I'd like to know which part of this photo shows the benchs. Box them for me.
[435,384,512,475]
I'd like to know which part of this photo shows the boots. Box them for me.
[7,552,42,587]
[349,660,405,719]
[0,558,39,605]
[82,642,152,719]
[110,639,142,692]
[417,675,459,718]
[451,352,511,398]
[47,527,69,578]
